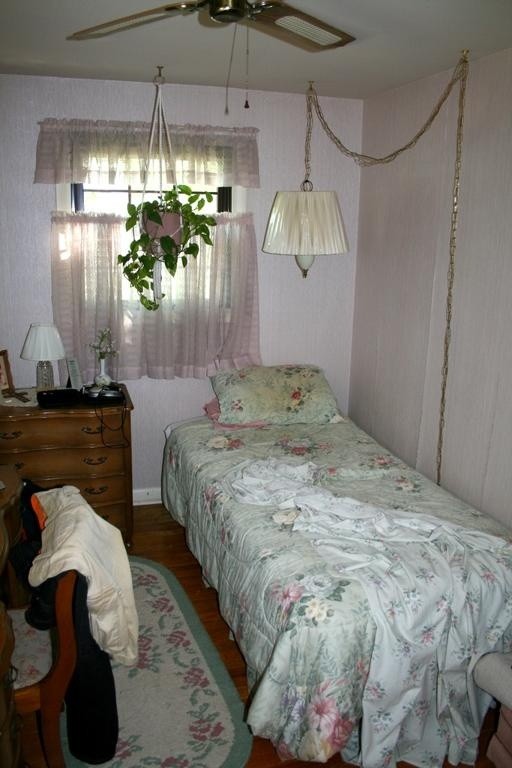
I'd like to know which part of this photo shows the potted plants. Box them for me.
[115,179,220,311]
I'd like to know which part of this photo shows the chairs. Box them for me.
[6,484,81,768]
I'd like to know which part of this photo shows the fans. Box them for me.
[65,0,358,56]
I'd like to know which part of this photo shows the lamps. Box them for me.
[261,84,349,280]
[20,322,67,392]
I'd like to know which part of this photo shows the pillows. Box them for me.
[200,362,340,430]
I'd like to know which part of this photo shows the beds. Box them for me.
[159,412,511,767]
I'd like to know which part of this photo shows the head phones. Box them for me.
[80,382,124,399]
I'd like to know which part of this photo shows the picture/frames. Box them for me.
[0,349,15,395]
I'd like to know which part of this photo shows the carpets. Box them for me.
[60,553,255,768]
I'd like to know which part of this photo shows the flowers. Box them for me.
[88,328,120,361]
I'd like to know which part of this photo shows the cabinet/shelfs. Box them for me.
[0,382,134,552]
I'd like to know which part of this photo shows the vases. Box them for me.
[94,358,113,387]
